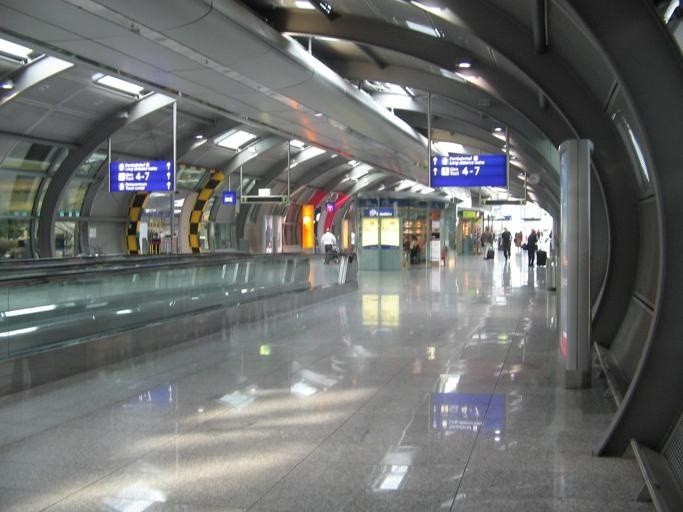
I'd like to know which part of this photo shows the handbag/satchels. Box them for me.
[521,241,528,250]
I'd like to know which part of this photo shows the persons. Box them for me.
[526,229,537,268]
[320,227,336,264]
[514,229,522,250]
[526,269,535,303]
[480,227,493,260]
[503,261,513,298]
[485,260,494,287]
[409,235,420,264]
[501,227,511,258]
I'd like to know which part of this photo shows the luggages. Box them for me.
[487,246,494,259]
[537,251,546,266]
